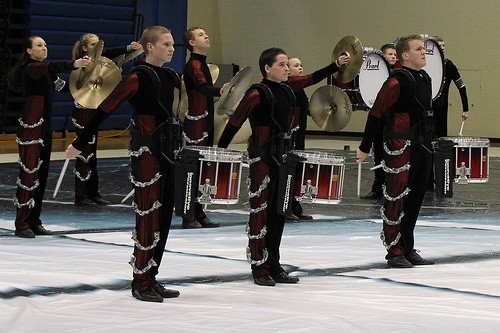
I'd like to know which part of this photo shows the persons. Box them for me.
[13,36,91,237]
[357,33,469,269]
[219,48,350,285]
[72,33,141,207]
[179,26,229,229]
[65,25,186,303]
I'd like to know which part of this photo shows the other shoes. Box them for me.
[200,217,219,228]
[75,198,97,207]
[284,214,299,223]
[31,224,53,235]
[273,271,299,283]
[388,255,413,268]
[181,219,202,229]
[14,227,35,238]
[360,190,383,199]
[297,212,313,221]
[154,283,180,298]
[90,196,112,206]
[405,249,434,265]
[254,273,276,286]
[132,285,163,302]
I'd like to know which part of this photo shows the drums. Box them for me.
[439,135,491,185]
[325,46,391,111]
[182,145,243,205]
[287,150,349,206]
[391,33,446,102]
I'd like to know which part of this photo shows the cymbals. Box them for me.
[308,84,354,133]
[117,45,145,65]
[207,63,220,84]
[217,66,253,116]
[76,39,105,89]
[69,54,121,109]
[331,35,364,83]
